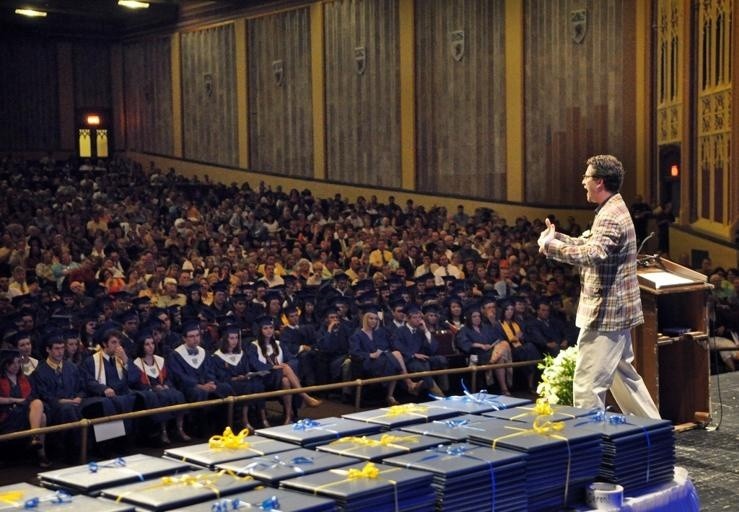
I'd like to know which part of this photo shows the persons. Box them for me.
[1,154,324,468]
[629,195,679,259]
[538,154,660,422]
[293,190,594,407]
[677,253,739,370]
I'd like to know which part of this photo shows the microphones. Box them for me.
[636,231,655,258]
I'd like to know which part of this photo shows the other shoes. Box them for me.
[341,380,537,405]
[31,395,323,468]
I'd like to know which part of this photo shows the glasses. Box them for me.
[582,174,597,179]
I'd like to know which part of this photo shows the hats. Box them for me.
[2,274,563,363]
